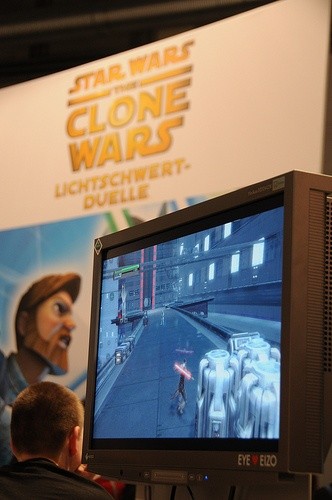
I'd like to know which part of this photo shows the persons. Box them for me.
[0,382,115,500]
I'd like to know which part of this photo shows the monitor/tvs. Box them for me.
[81,171,332,484]
